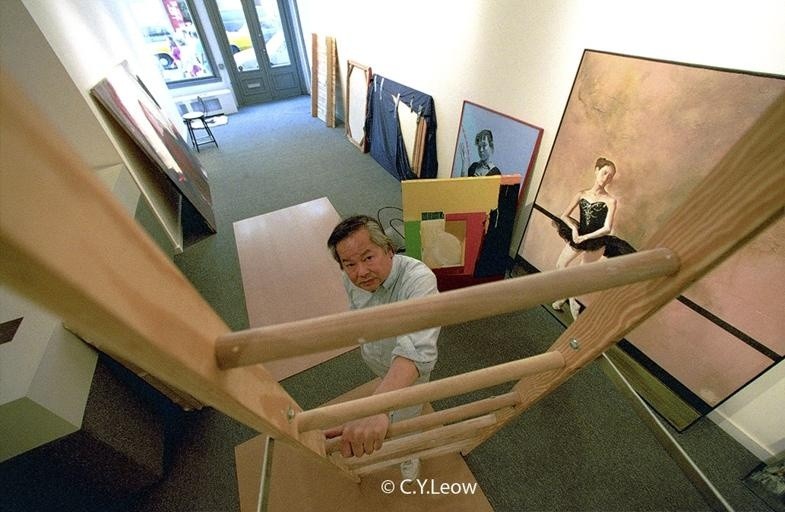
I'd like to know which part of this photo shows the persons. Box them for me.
[551,157,618,320]
[137,99,211,206]
[459,129,505,177]
[319,214,444,485]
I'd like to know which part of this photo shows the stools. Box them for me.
[183,110,219,153]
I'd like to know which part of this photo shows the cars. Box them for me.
[149,19,293,73]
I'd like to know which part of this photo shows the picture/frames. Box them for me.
[344,61,430,186]
[507,48,785,434]
[448,99,544,209]
[141,0,223,89]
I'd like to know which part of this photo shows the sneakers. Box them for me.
[552,299,581,320]
[400,459,421,484]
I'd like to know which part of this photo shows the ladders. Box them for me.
[0,68,784,488]
